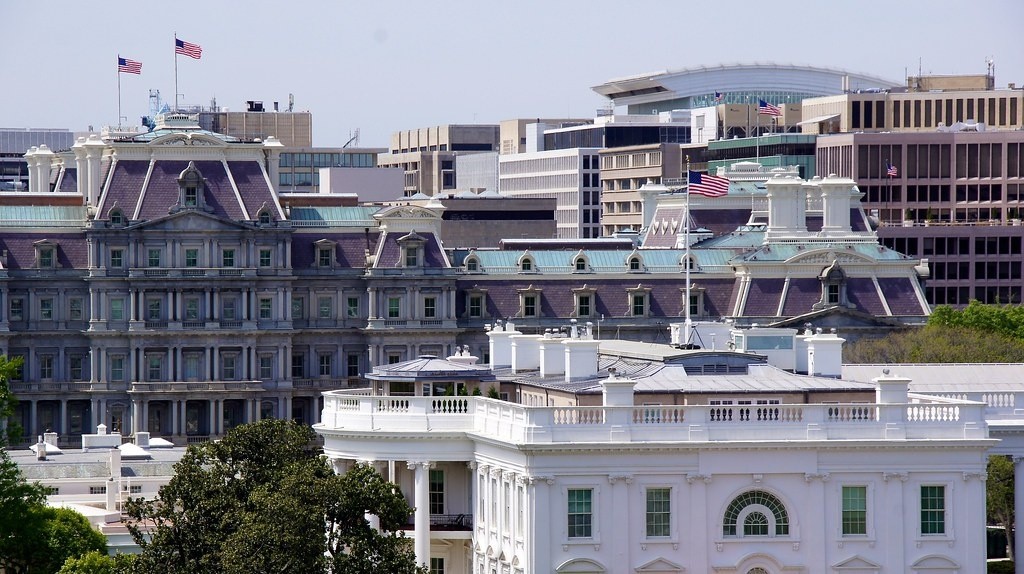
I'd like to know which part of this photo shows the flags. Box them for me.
[118,58,142,75]
[760,100,781,116]
[716,92,722,100]
[689,170,729,198]
[176,39,202,59]
[886,162,897,175]
